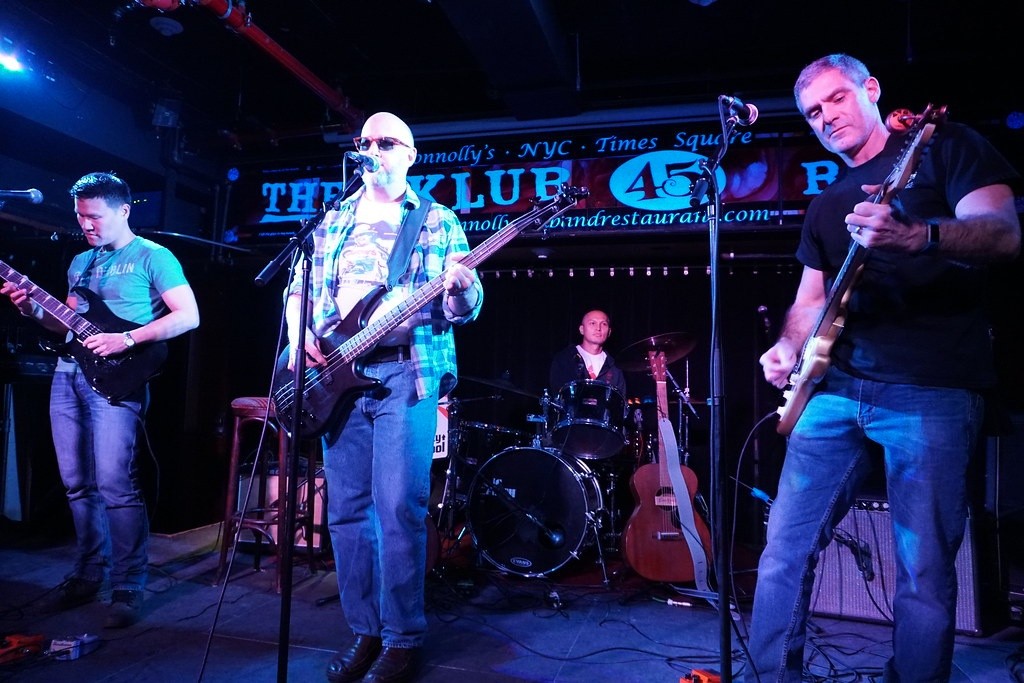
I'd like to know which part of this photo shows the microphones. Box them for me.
[0,187,44,204]
[851,540,873,580]
[526,513,564,547]
[346,150,379,173]
[718,95,759,126]
[579,356,591,380]
[634,408,643,431]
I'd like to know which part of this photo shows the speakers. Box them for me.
[981,412,1024,601]
[809,499,999,638]
[235,462,334,560]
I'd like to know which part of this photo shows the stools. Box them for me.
[211,396,317,591]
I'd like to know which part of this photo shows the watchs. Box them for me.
[921,218,939,256]
[123,331,135,348]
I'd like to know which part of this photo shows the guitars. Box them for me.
[268,179,592,442]
[772,97,957,436]
[0,257,171,403]
[618,350,714,584]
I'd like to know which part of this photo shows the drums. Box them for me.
[446,418,540,502]
[465,445,603,579]
[547,378,629,461]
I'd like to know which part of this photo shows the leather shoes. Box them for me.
[365,642,422,683]
[326,630,385,679]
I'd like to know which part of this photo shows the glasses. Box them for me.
[353,136,412,151]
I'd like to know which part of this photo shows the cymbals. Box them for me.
[669,396,706,405]
[614,331,699,372]
[456,373,541,404]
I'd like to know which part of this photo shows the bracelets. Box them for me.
[21,305,40,317]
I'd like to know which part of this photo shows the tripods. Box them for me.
[594,472,623,557]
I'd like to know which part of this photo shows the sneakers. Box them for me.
[41,576,111,607]
[111,587,145,615]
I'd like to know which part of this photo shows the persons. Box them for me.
[1,171,199,619]
[542,310,626,407]
[283,112,485,682]
[744,52,1023,683]
